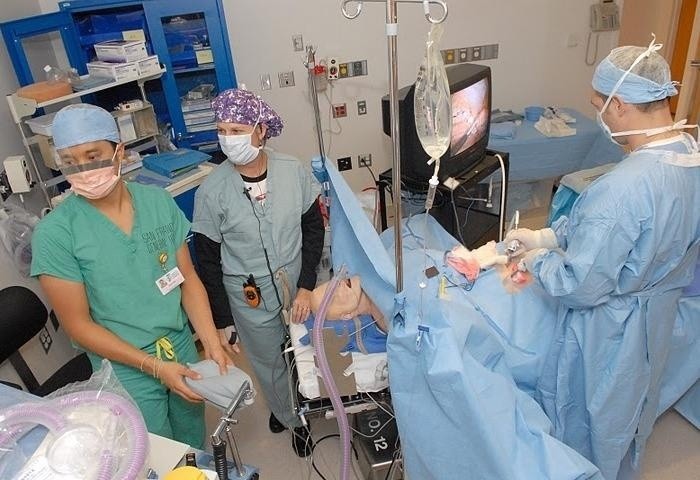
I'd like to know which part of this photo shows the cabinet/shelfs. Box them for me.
[0,0,238,166]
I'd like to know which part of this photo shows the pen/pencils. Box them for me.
[243,273,254,287]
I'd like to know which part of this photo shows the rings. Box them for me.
[302,307,308,312]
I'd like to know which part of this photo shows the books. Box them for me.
[181,81,218,134]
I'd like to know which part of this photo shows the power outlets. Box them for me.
[359,155,372,167]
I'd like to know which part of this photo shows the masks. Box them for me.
[216,124,261,166]
[593,97,620,148]
[57,145,121,202]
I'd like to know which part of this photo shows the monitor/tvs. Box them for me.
[381,63,491,183]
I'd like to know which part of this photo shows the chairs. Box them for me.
[0,383,252,480]
[0,286,91,396]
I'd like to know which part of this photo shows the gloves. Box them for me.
[502,225,560,259]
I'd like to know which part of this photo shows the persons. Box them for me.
[29,102,238,451]
[305,274,391,335]
[191,87,327,458]
[501,44,700,480]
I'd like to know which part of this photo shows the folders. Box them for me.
[142,148,212,179]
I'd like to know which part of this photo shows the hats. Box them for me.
[49,101,121,153]
[210,88,284,139]
[590,44,678,104]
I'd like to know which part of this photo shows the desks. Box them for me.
[488,107,604,182]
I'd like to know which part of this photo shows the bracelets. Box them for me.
[139,354,164,387]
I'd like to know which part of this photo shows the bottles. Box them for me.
[192,35,211,51]
[43,65,72,94]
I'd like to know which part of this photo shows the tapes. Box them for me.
[229,332,236,344]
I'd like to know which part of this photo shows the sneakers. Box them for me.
[268,410,286,434]
[291,413,312,459]
